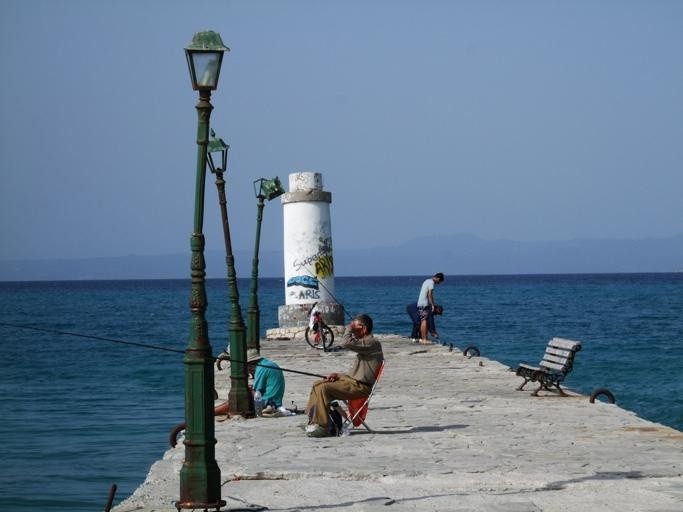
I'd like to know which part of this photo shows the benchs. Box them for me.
[515,335,583,396]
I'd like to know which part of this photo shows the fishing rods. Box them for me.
[303,266,354,319]
[2,324,329,379]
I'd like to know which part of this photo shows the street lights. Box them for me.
[245,174,284,352]
[170,25,229,512]
[202,125,258,422]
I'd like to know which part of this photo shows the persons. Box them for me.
[301,315,382,437]
[215,349,284,415]
[407,303,443,343]
[417,273,444,344]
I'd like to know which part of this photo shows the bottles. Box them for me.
[288,400,297,415]
[254,389,262,417]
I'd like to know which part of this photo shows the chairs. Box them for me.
[330,359,388,439]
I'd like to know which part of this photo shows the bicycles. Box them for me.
[302,301,334,353]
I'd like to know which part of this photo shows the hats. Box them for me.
[247,348,262,364]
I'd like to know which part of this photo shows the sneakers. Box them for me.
[307,426,332,437]
